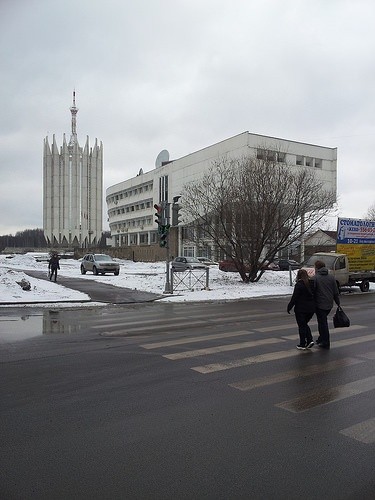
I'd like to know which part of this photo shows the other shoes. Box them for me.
[55,279,57,282]
[306,340,314,348]
[297,345,306,349]
[316,340,329,350]
[50,277,52,281]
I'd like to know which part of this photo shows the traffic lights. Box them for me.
[173,195,181,202]
[172,203,183,226]
[159,230,167,248]
[153,201,165,226]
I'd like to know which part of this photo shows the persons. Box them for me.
[48,253,60,282]
[287,269,317,350]
[310,261,342,350]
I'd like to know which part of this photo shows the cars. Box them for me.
[197,257,219,265]
[219,257,301,273]
[171,256,208,272]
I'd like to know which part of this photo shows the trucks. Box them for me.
[300,252,375,292]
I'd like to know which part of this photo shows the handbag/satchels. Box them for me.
[333,306,350,328]
[47,269,50,278]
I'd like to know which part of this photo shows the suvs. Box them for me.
[80,253,120,276]
